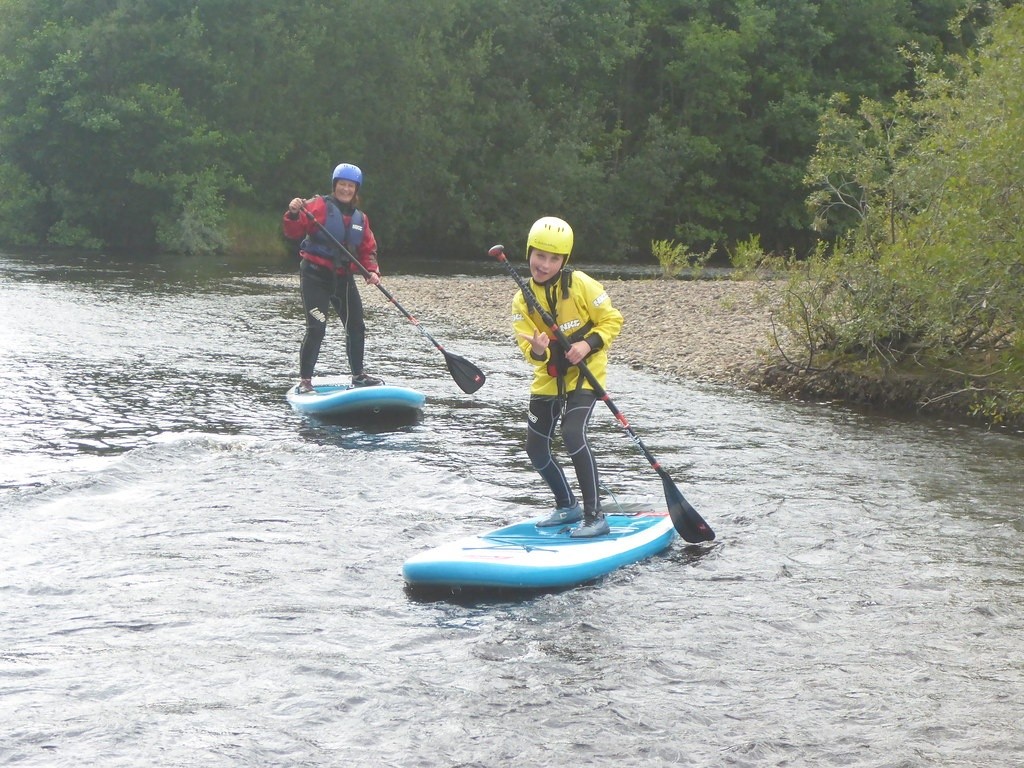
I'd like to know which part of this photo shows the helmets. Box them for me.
[332,163,363,193]
[526,216,574,269]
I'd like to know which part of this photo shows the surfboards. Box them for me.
[401,508,683,594]
[285,374,432,419]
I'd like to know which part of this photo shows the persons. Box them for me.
[282,162,385,395]
[511,215,624,538]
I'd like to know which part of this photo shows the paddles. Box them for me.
[490,240,718,546]
[295,194,487,394]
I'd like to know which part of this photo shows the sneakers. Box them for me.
[294,379,317,394]
[570,510,611,538]
[535,504,583,528]
[348,374,385,386]
